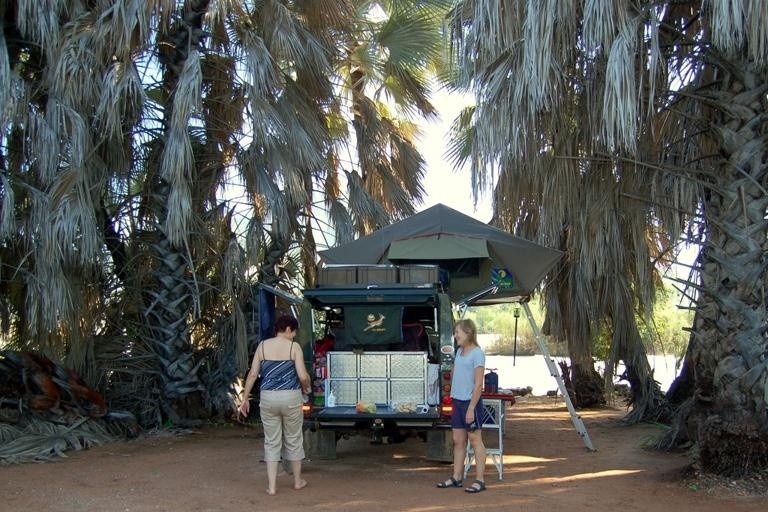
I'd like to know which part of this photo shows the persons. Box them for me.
[436,318,486,492]
[240,315,312,495]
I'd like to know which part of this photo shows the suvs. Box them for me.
[255,263,500,464]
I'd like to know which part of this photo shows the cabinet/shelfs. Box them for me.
[463,399,505,480]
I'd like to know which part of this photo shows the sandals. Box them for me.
[465,480,486,492]
[436,476,464,488]
